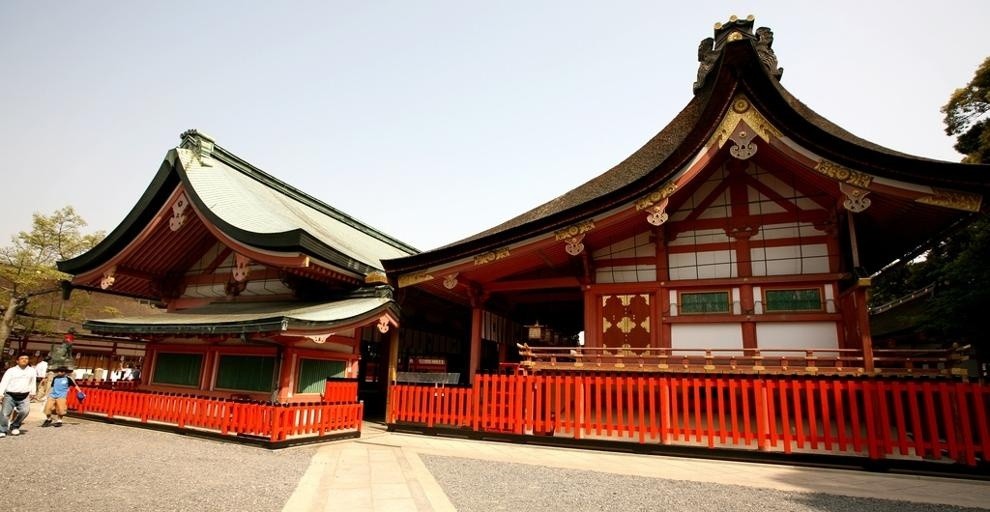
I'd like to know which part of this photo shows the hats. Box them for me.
[49,367,73,373]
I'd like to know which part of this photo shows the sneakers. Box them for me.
[0,428,20,438]
[41,419,63,427]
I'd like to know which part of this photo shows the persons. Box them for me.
[131,365,142,380]
[0,353,86,438]
[121,361,132,380]
